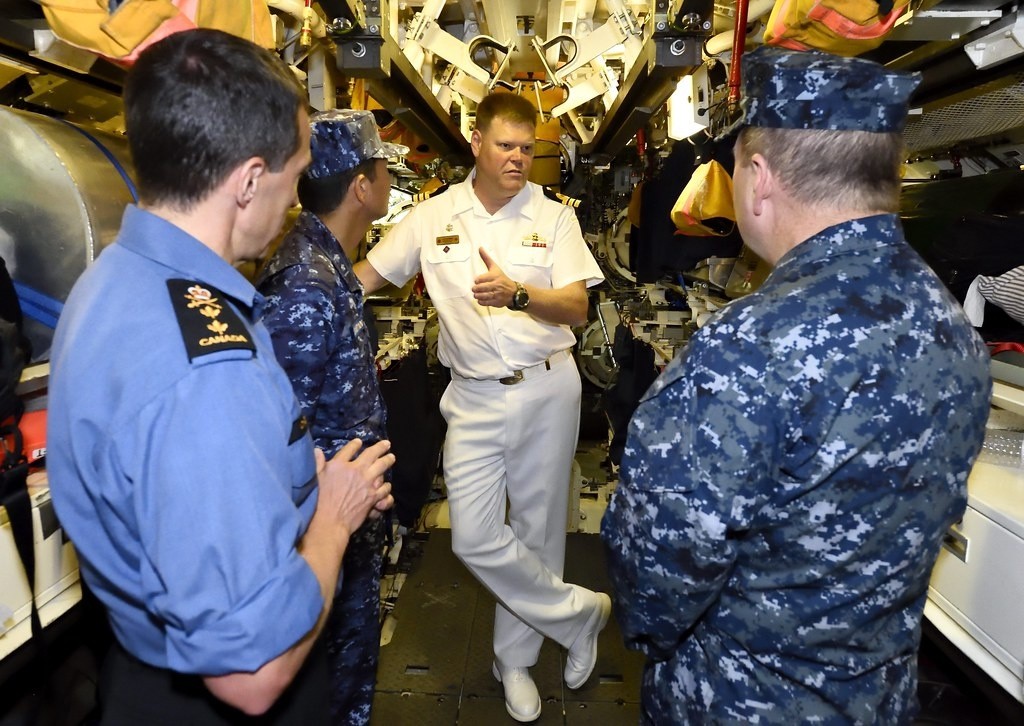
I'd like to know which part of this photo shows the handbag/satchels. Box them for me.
[764,0,941,59]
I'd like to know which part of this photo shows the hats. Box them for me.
[742,46,923,132]
[305,108,410,178]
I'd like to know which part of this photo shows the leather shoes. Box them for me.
[492,657,542,722]
[564,592,611,689]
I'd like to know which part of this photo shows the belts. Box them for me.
[450,349,570,386]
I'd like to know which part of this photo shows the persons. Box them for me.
[254,107,409,726]
[48,28,396,726]
[599,48,994,725]
[353,92,612,721]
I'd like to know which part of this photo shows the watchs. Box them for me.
[506,280,530,310]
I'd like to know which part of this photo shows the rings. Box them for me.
[494,290,496,299]
